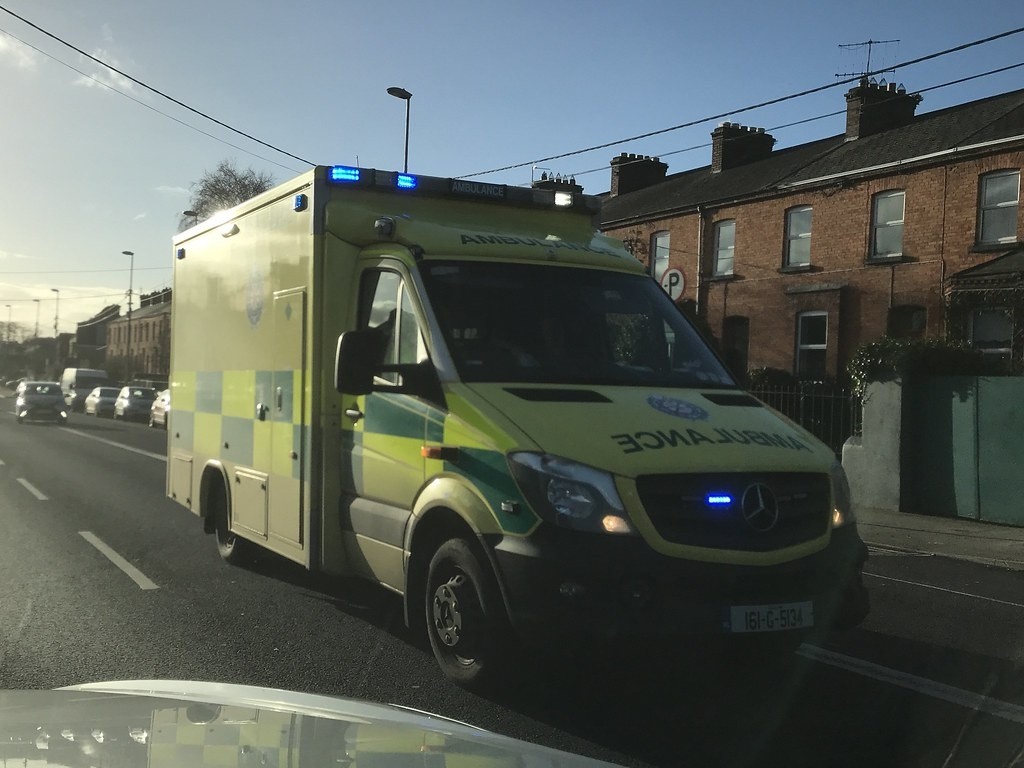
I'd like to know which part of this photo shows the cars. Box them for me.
[113,386,159,419]
[13,381,66,425]
[83,386,120,417]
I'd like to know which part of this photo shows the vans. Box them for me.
[60,367,110,410]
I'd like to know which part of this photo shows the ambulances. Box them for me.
[166,165,872,690]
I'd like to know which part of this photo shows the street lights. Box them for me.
[387,85,414,173]
[122,250,133,376]
[183,211,199,226]
